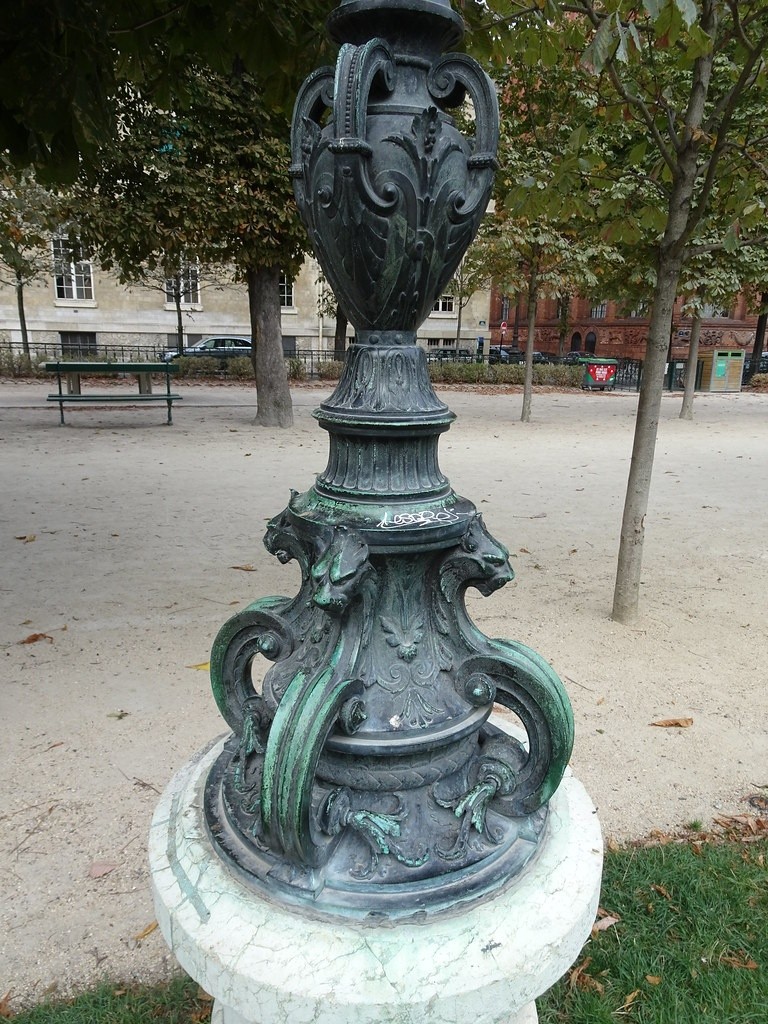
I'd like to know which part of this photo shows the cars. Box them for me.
[563,351,598,366]
[744,352,767,373]
[478,348,561,365]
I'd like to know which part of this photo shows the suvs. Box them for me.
[158,335,254,363]
[425,347,476,363]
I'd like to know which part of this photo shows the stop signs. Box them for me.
[500,322,508,334]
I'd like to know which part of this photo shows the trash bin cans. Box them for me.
[698,349,745,393]
[578,357,618,391]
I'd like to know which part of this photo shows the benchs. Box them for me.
[46,361,183,425]
